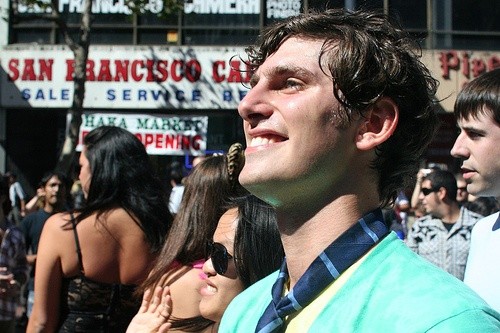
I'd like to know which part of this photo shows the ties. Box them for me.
[254,208,389,333]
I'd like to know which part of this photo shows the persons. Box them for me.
[0,8,500,333]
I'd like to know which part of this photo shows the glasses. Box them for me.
[205,238,244,275]
[421,186,435,196]
